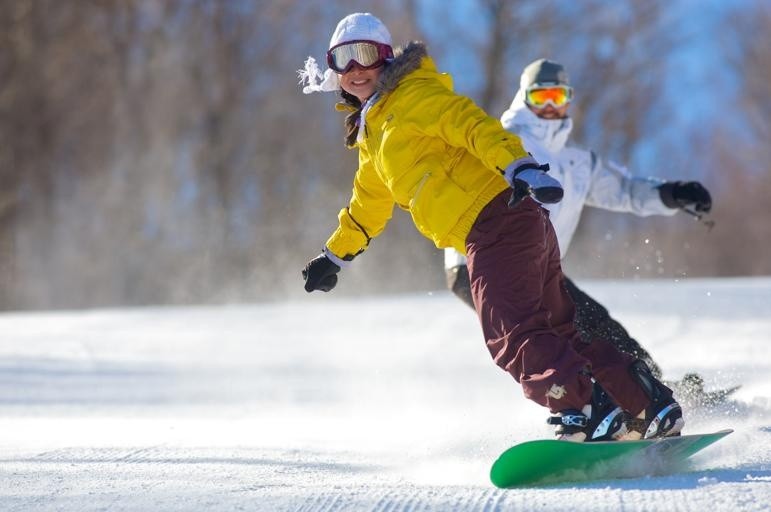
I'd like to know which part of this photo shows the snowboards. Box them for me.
[490,428,736,487]
[667,373,743,410]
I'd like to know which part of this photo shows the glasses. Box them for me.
[522,82,573,109]
[326,40,393,74]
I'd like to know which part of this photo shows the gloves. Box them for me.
[504,156,564,209]
[303,255,340,292]
[671,181,711,213]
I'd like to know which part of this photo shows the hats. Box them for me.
[297,13,394,94]
[521,60,566,92]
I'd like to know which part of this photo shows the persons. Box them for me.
[444,59,712,438]
[301,12,684,446]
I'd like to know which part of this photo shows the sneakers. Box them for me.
[623,364,684,440]
[547,374,623,442]
